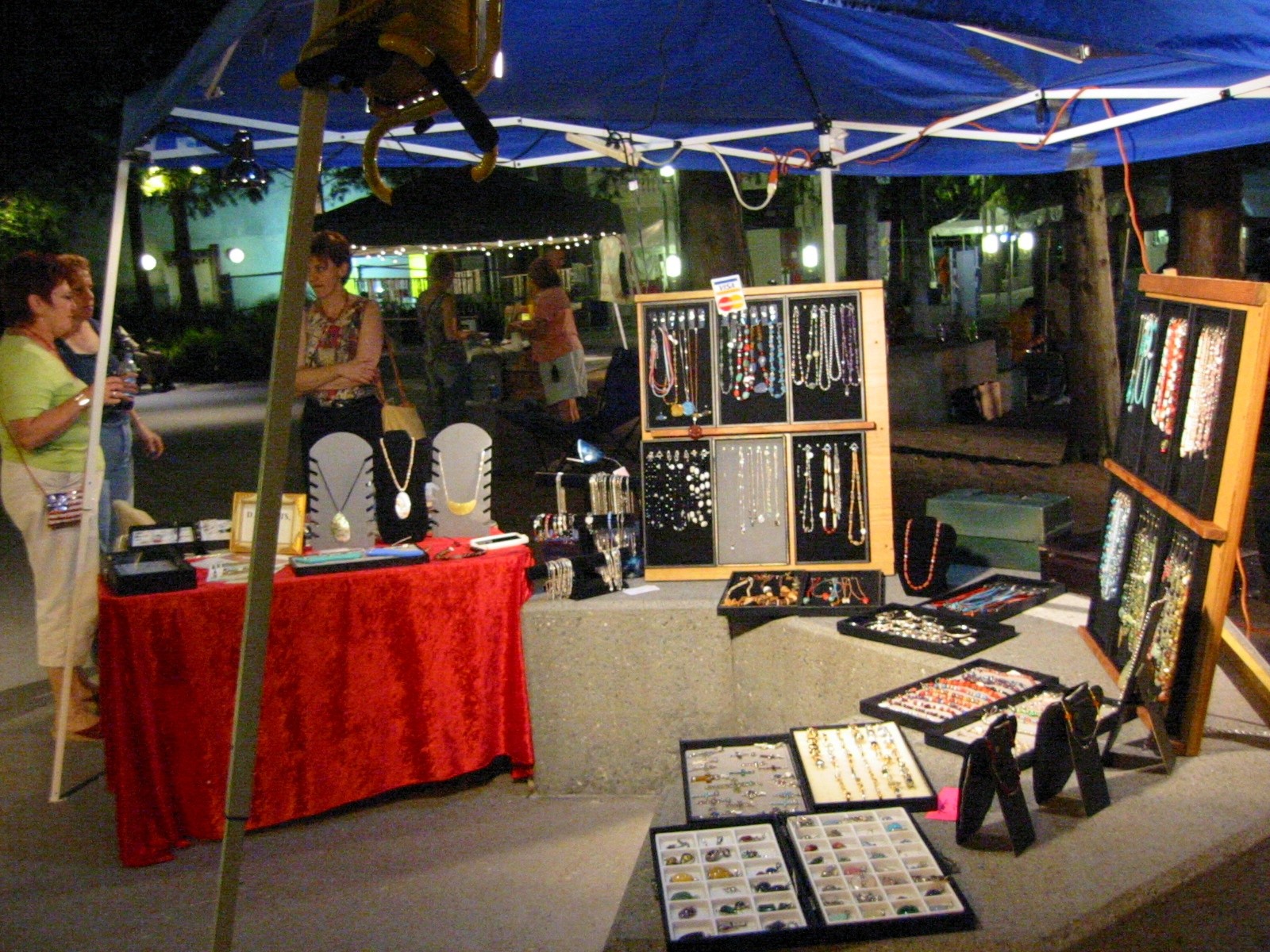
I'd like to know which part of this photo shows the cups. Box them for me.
[511,332,521,348]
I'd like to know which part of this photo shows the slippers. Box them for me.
[82,690,101,710]
[51,715,103,742]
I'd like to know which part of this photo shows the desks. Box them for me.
[79,531,553,872]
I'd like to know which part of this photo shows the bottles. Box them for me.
[114,349,137,410]
[969,320,977,342]
[936,324,945,343]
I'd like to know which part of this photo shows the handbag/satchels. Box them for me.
[381,399,426,440]
[47,489,84,529]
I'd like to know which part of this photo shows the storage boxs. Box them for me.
[926,487,1075,574]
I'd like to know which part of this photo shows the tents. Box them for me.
[43,0,1269,952]
[927,159,1269,353]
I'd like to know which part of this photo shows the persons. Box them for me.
[506,255,587,449]
[415,251,472,427]
[0,259,109,744]
[1005,262,1075,406]
[50,255,163,558]
[293,229,383,516]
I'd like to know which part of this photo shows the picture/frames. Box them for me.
[228,490,310,554]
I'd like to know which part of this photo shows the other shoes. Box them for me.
[1051,395,1071,405]
[1029,393,1050,402]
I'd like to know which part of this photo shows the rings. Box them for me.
[112,390,117,397]
[652,719,963,942]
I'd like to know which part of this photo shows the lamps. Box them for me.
[220,131,268,190]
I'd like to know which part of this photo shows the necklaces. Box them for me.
[15,324,59,351]
[311,303,1234,705]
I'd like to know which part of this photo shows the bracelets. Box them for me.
[130,416,142,426]
[71,393,93,412]
